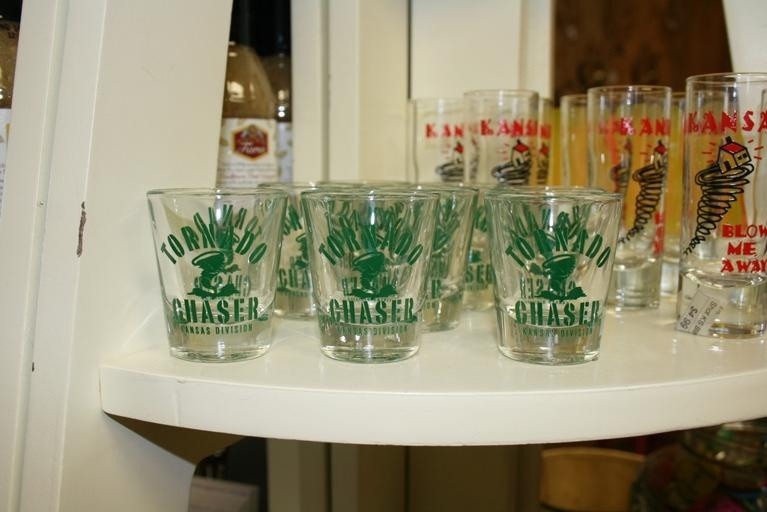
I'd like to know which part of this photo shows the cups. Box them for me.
[406,70,767,341]
[146,187,289,364]
[252,179,626,366]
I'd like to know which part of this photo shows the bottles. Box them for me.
[213,0,293,216]
